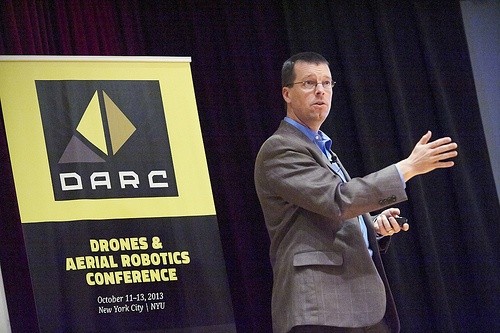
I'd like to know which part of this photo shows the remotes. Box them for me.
[389,217,408,228]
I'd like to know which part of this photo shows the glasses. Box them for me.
[286,79,336,89]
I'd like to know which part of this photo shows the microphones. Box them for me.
[332,156,336,163]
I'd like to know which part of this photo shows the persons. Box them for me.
[254,52,458,333]
